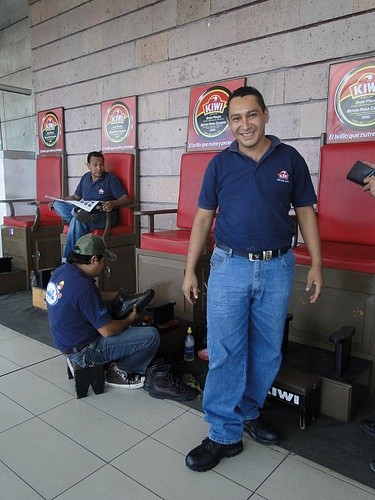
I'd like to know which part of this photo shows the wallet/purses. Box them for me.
[345,160,375,187]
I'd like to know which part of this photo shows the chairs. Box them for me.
[104,151,219,357]
[265,133,375,424]
[0,153,69,296]
[30,149,140,312]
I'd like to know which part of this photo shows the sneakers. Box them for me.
[104,362,145,390]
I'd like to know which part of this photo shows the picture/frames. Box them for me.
[185,77,247,153]
[101,95,138,152]
[38,106,66,154]
[325,55,375,145]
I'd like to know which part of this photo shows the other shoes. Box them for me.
[108,288,155,321]
[75,212,100,225]
[149,364,201,401]
[359,419,375,438]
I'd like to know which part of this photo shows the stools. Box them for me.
[66,357,110,400]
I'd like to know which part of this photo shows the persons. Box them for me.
[47,151,130,275]
[45,233,161,389]
[362,160,375,197]
[182,85,324,473]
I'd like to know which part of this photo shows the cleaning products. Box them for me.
[184,327,194,362]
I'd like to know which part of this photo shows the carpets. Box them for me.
[0,288,375,490]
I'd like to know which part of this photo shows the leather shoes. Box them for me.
[245,417,282,447]
[185,437,243,471]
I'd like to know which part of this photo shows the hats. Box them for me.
[73,232,118,262]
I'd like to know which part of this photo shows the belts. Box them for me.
[216,241,292,262]
